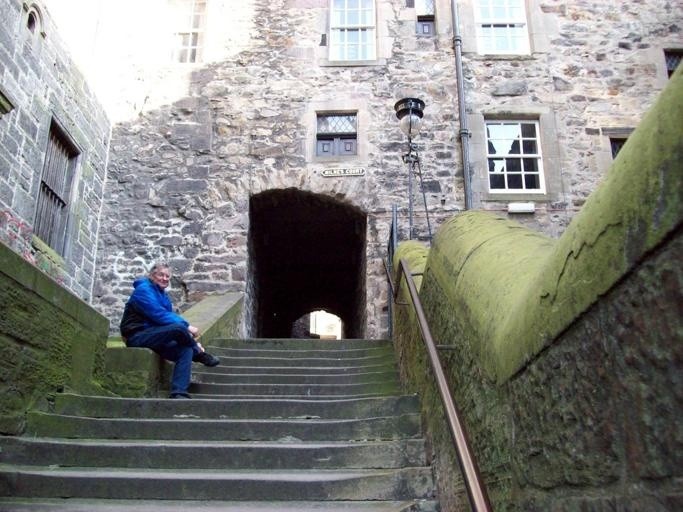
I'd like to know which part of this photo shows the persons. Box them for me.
[118,261,222,399]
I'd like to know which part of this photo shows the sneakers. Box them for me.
[192,353,219,367]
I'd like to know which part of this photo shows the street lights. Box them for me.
[394,95,424,241]
